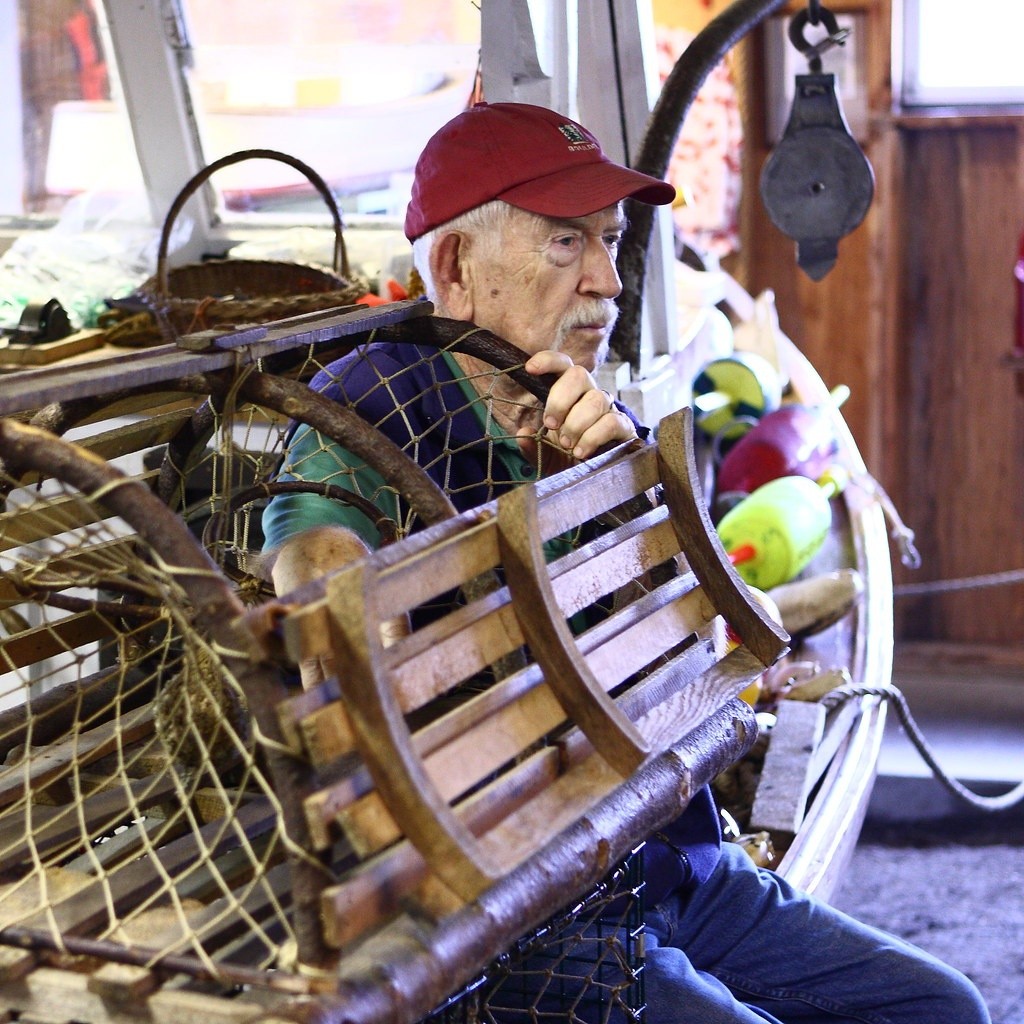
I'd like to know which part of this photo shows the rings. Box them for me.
[603,390,615,412]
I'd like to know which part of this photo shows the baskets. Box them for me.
[136,148,370,344]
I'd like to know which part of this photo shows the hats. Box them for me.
[405,101,676,244]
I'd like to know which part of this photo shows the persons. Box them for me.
[261,102,991,1023]
